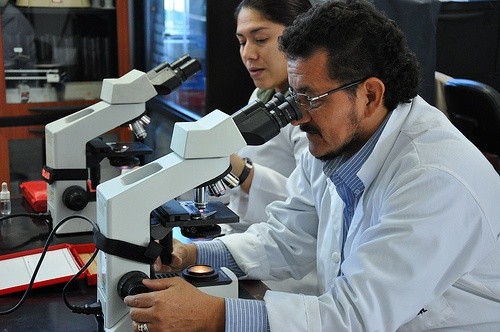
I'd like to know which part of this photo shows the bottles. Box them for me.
[0,181,11,214]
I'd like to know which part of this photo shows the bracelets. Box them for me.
[236,157,254,185]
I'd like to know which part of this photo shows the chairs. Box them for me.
[440,80,499,169]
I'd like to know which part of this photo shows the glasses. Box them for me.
[288,75,375,111]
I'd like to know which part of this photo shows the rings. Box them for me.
[138,323,149,332]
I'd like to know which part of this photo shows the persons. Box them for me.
[0,1,37,68]
[124,2,500,332]
[219,1,327,297]
